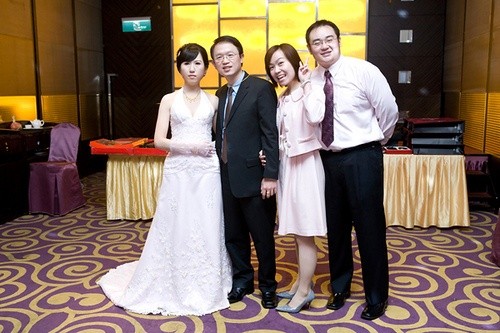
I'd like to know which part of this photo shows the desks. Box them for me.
[105,141,470,229]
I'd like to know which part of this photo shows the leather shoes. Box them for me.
[227,286,255,303]
[327,289,351,309]
[361,299,389,320]
[261,291,278,307]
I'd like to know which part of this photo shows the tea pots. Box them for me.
[30,118,45,128]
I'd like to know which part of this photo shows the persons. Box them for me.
[305,19,399,321]
[98,41,233,318]
[257,42,328,314]
[210,35,280,308]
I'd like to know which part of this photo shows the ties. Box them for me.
[321,70,335,147]
[221,87,234,163]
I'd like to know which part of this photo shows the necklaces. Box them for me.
[181,90,200,102]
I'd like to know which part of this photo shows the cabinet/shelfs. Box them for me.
[0,120,58,224]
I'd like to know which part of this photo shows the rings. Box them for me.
[267,192,270,194]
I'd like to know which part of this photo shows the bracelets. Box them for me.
[301,80,311,88]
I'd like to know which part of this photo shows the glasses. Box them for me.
[309,36,341,49]
[214,51,242,63]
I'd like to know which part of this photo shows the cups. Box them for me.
[25,125,32,128]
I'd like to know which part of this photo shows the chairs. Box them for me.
[27,122,89,216]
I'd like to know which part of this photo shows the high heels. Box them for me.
[276,280,314,298]
[275,289,315,313]
